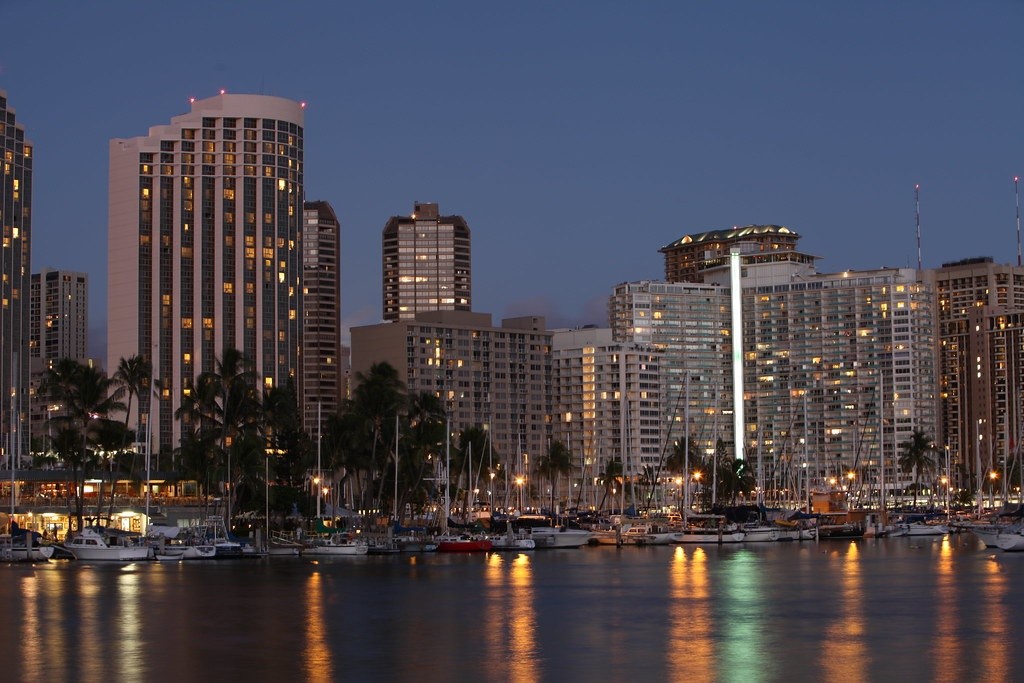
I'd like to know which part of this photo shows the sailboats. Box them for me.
[266,398,589,555]
[0,408,265,561]
[590,367,1024,551]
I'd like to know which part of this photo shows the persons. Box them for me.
[114,492,168,507]
[200,494,214,504]
[51,523,59,542]
[37,490,77,505]
[295,525,302,539]
[43,529,48,541]
